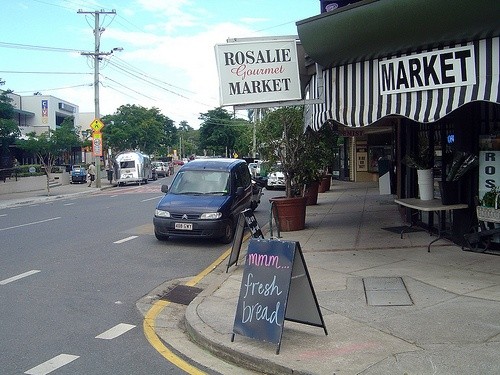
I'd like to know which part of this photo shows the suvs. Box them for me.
[248,159,290,191]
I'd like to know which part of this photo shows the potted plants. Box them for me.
[255,108,337,232]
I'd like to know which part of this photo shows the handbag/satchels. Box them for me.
[91,175,95,181]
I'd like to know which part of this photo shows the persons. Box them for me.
[88,162,96,187]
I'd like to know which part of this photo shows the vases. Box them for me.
[438,181,460,205]
[417,168,434,201]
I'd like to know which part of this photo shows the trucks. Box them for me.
[159,156,175,174]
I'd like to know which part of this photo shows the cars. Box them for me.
[173,160,185,166]
[151,163,158,181]
[152,161,170,177]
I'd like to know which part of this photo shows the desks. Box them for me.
[394,198,469,253]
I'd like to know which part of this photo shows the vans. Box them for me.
[152,159,253,244]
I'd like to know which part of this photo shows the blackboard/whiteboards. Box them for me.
[233,236,329,344]
[224,208,266,267]
